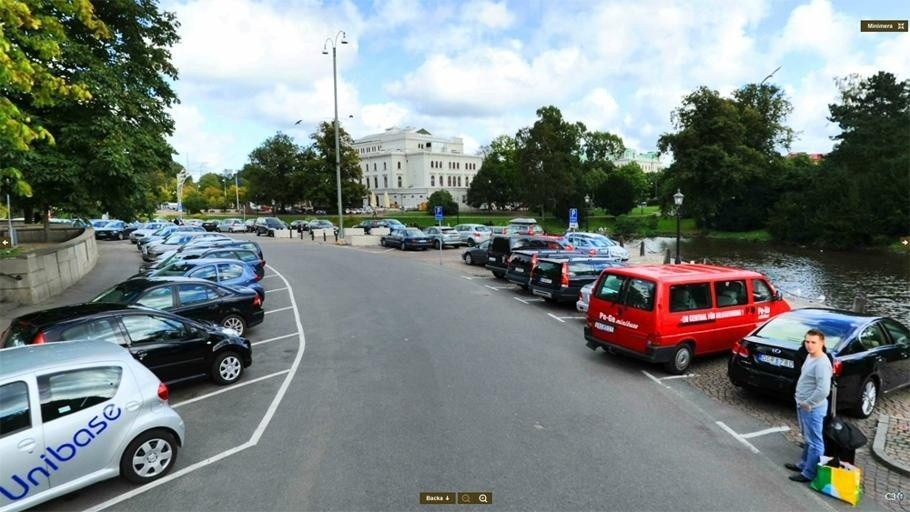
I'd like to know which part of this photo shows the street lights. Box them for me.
[221,178,227,199]
[673,189,685,264]
[583,193,590,232]
[322,29,349,245]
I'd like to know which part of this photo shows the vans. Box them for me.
[584,262,791,374]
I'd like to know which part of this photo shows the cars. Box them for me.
[3,302,254,393]
[0,337,185,512]
[11,217,267,337]
[182,218,341,238]
[462,232,653,313]
[726,306,909,419]
[352,218,544,251]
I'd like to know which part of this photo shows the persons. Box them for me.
[784,329,834,483]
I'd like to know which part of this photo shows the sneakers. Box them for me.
[785,463,803,472]
[788,473,813,483]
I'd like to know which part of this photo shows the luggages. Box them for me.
[823,381,866,465]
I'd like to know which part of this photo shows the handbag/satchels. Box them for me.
[809,455,864,506]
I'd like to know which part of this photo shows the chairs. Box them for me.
[673,288,697,310]
[718,285,739,306]
